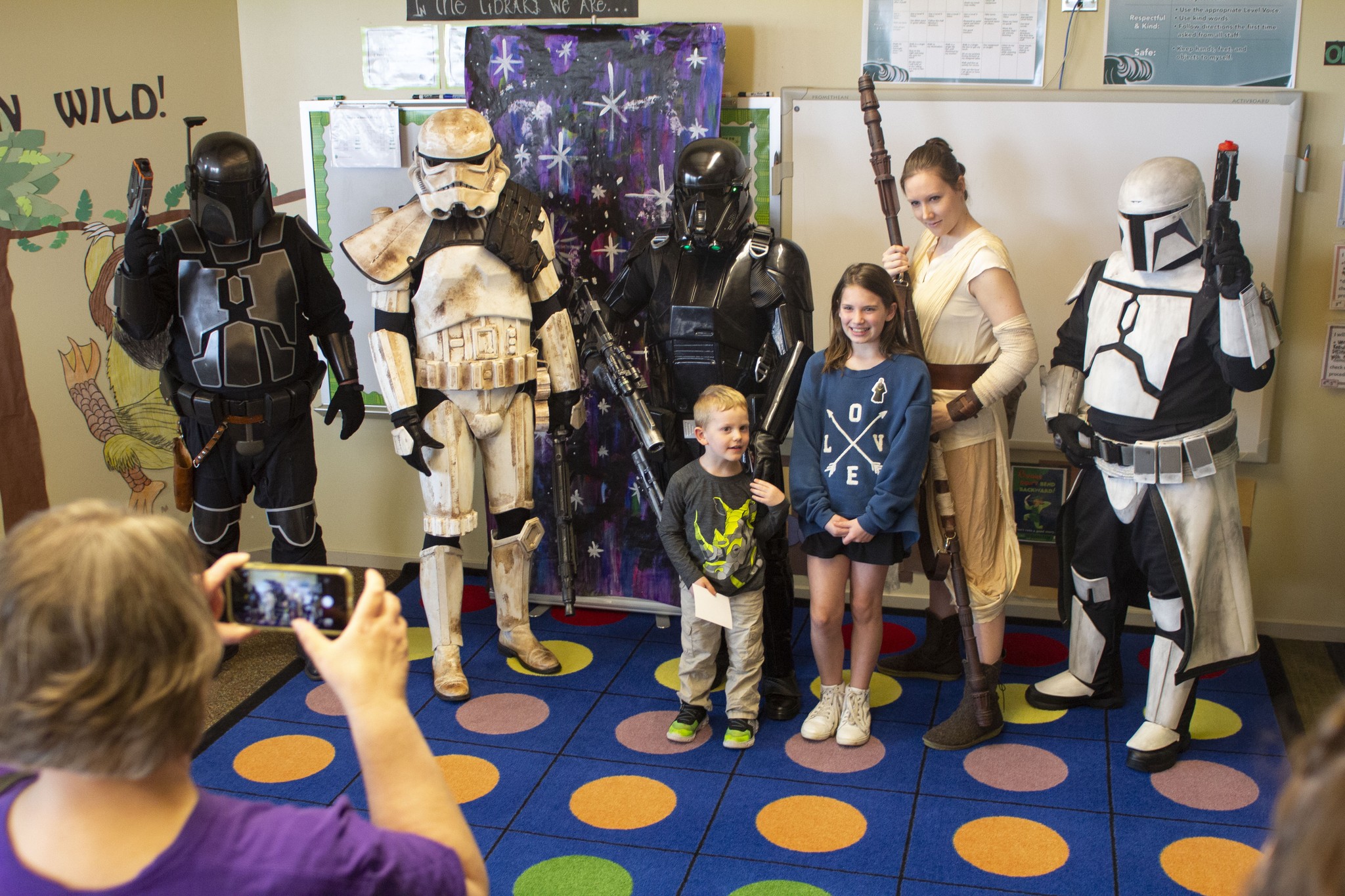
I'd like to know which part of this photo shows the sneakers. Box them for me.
[723,717,759,748]
[666,700,710,743]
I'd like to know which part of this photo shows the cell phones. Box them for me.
[222,561,353,637]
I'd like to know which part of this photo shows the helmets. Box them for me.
[1118,156,1213,272]
[185,131,273,240]
[676,137,749,254]
[407,107,511,221]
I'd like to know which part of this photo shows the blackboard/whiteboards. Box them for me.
[779,86,1304,465]
[297,99,781,422]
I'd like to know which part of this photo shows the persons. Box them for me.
[335,111,585,703]
[1249,685,1345,896]
[592,134,812,725]
[884,136,1041,753]
[0,495,490,896]
[238,571,324,626]
[659,383,787,751]
[790,261,932,747]
[115,133,364,663]
[1027,158,1284,773]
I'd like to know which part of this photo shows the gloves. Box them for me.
[124,212,164,275]
[749,431,785,494]
[584,353,613,390]
[546,391,586,438]
[1203,216,1254,300]
[1049,414,1095,468]
[390,407,444,476]
[325,383,366,439]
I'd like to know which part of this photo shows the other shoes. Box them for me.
[763,679,801,720]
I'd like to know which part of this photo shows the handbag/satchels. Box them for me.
[172,434,194,512]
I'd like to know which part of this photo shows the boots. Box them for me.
[835,682,873,744]
[925,656,1004,750]
[800,681,847,741]
[1123,591,1193,771]
[878,603,962,681]
[489,516,562,674]
[419,545,470,702]
[1024,561,1126,709]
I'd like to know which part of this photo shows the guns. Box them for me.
[552,425,580,618]
[568,275,666,523]
[126,158,160,266]
[1200,140,1240,300]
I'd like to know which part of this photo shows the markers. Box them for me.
[738,91,774,97]
[412,94,466,99]
[773,150,779,166]
[1303,144,1311,161]
[313,96,346,100]
[722,92,735,98]
